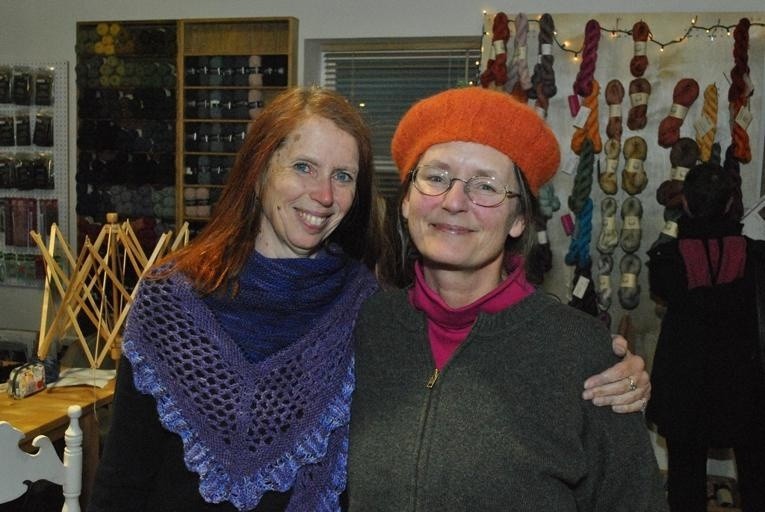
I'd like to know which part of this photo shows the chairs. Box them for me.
[0,403,84,512]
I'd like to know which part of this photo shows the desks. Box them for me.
[0,379,116,512]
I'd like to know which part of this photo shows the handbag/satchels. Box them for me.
[8,362,47,400]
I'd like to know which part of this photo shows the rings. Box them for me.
[629,376,635,391]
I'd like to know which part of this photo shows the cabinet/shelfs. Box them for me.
[76,15,299,323]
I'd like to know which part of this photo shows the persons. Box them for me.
[644,160,764,512]
[88,89,651,511]
[348,86,668,511]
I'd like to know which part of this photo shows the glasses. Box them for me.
[409,164,525,210]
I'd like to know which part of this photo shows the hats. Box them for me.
[391,87,560,199]
